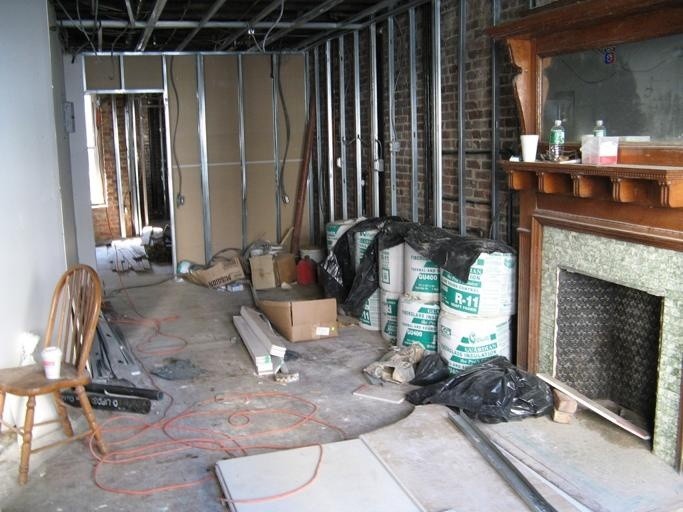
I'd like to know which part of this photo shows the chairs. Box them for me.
[1,263,109,484]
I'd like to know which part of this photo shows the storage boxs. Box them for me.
[258,289,337,343]
[185,247,305,288]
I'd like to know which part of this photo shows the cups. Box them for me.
[40,346,62,380]
[519,134,539,163]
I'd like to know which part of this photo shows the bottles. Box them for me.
[548,120,565,162]
[593,120,606,138]
